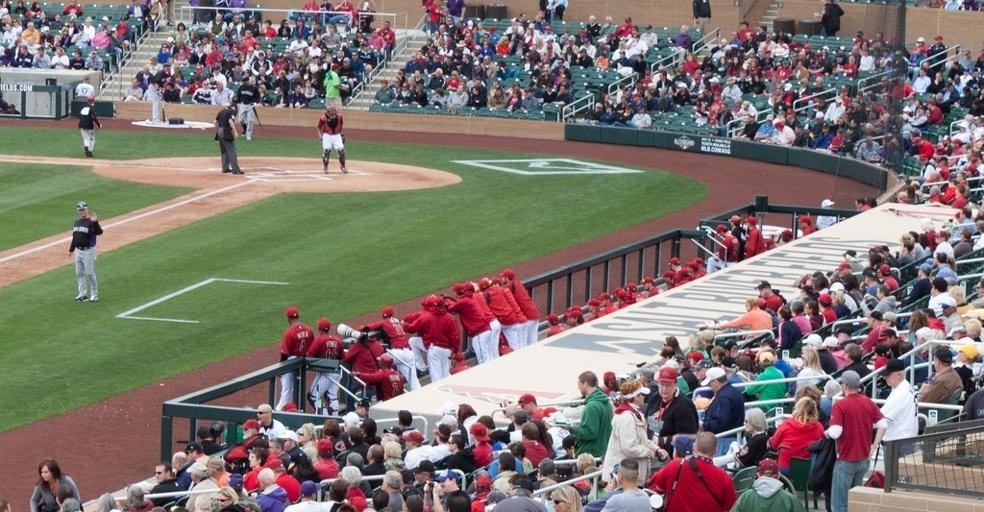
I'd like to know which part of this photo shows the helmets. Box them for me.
[325,106,337,119]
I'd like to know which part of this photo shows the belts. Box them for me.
[77,246,90,250]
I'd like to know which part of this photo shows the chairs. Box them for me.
[2,1,157,83]
[371,1,982,178]
[897,242,984,327]
[123,2,398,110]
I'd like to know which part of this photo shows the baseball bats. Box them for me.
[251,103,264,131]
[160,97,166,122]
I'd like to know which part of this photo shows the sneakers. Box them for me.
[341,167,348,173]
[222,168,245,175]
[241,129,252,143]
[323,167,328,174]
[90,294,100,302]
[74,294,89,302]
[83,146,92,158]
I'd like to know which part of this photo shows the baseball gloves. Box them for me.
[341,132,345,145]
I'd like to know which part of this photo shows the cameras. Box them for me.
[428,482,434,488]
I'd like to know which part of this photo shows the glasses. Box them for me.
[217,499,231,502]
[153,472,163,475]
[554,499,567,504]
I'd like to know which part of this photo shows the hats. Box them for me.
[0,0,388,112]
[623,383,651,399]
[663,211,984,367]
[299,480,322,497]
[699,366,726,387]
[316,318,331,330]
[382,425,401,437]
[876,358,906,378]
[77,201,88,212]
[553,463,572,482]
[357,265,656,369]
[657,366,678,382]
[515,393,538,407]
[286,306,298,317]
[757,456,779,475]
[511,476,534,492]
[433,469,460,482]
[820,199,836,209]
[433,414,459,440]
[392,0,984,146]
[180,420,299,492]
[837,370,862,389]
[556,435,578,450]
[401,431,425,444]
[670,436,693,457]
[342,412,360,425]
[315,438,334,453]
[468,421,490,441]
